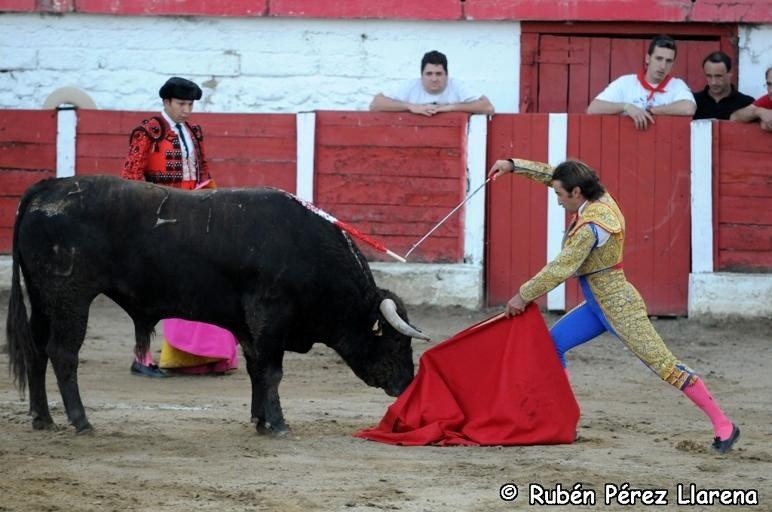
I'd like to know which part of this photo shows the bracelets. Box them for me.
[646,105,653,116]
[623,103,629,112]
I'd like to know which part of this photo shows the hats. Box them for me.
[158,76,203,100]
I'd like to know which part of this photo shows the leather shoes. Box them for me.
[129,361,176,380]
[711,423,740,454]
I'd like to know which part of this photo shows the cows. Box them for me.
[6,174,430,439]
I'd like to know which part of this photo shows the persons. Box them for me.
[729,66,771,132]
[368,48,497,117]
[692,51,755,120]
[585,35,696,132]
[120,75,214,379]
[487,155,744,455]
